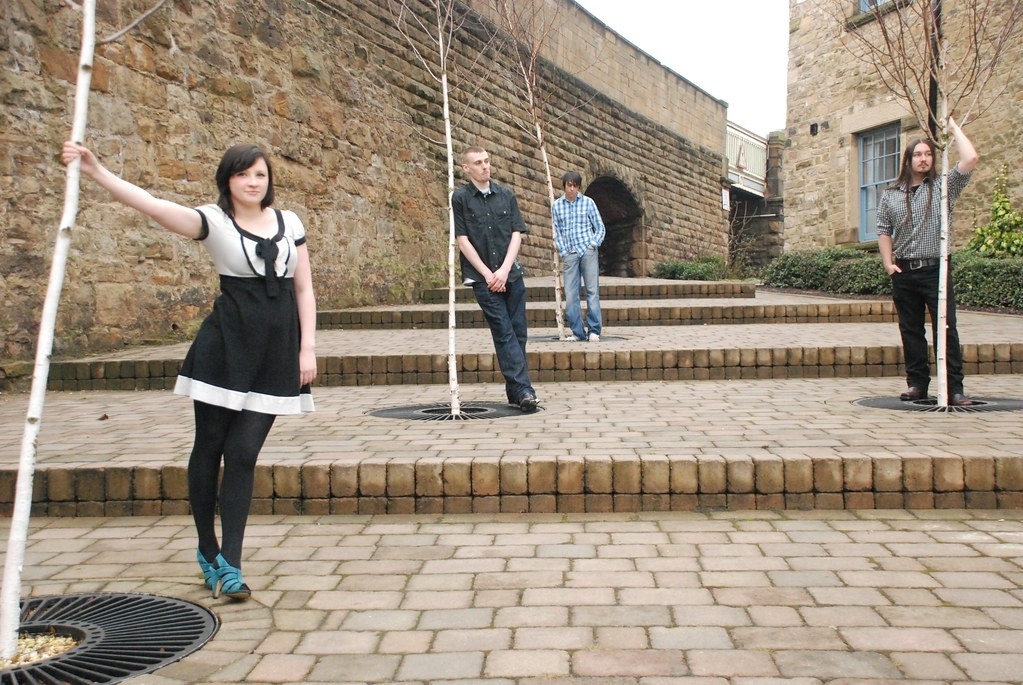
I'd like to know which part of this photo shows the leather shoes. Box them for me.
[900,387,928,400]
[951,394,972,405]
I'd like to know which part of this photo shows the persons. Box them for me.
[551,171,608,345]
[452,145,544,414]
[62,140,321,604]
[874,114,981,410]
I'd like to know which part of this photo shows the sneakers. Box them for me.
[588,333,599,342]
[509,392,542,412]
[559,335,581,342]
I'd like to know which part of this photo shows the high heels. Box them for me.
[210,553,251,598]
[196,546,220,589]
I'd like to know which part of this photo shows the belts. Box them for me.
[895,259,936,270]
